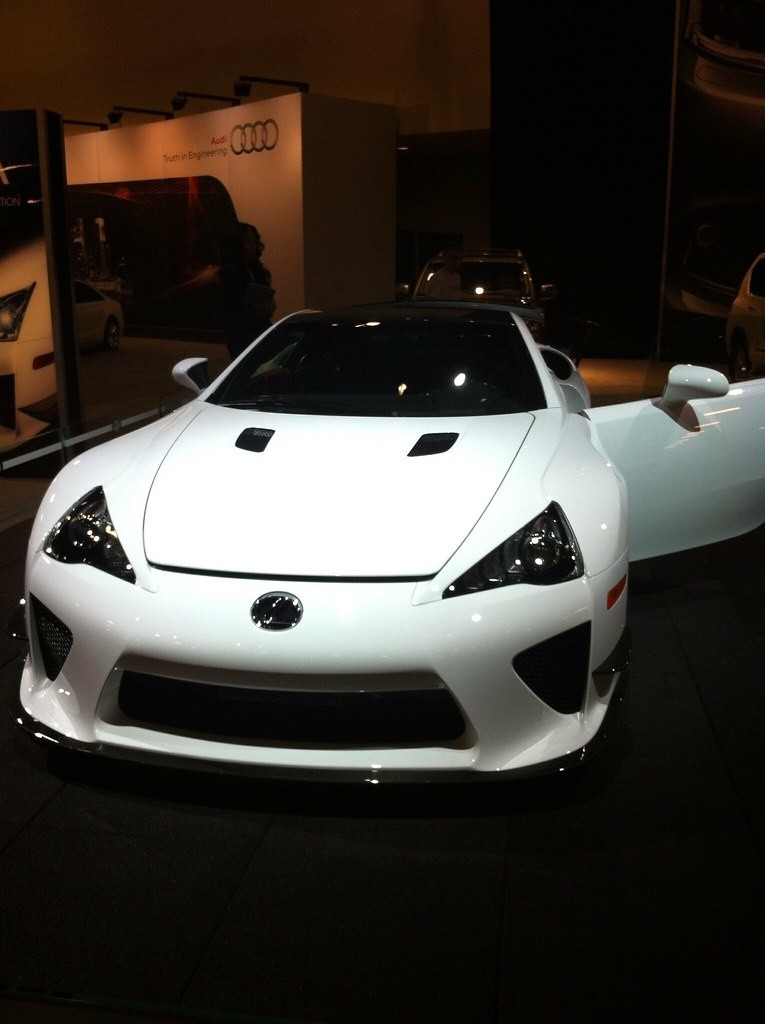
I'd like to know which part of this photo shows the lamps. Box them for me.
[232,75,309,98]
[171,91,240,112]
[106,106,175,123]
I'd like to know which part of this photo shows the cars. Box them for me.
[724,252,765,382]
[69,276,135,352]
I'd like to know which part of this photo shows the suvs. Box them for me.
[395,248,560,348]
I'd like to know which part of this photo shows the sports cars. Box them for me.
[1,283,765,789]
